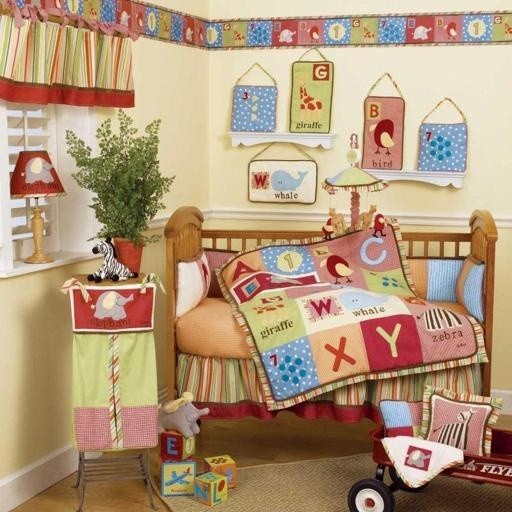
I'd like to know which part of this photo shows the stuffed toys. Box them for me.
[158,390,212,440]
[86,236,137,284]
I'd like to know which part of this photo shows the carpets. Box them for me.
[148,452,512,512]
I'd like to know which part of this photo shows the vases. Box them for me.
[64,108,177,277]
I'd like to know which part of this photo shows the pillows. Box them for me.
[417,384,503,457]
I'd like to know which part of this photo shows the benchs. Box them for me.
[162,203,498,402]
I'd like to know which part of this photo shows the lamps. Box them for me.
[9,149,67,264]
